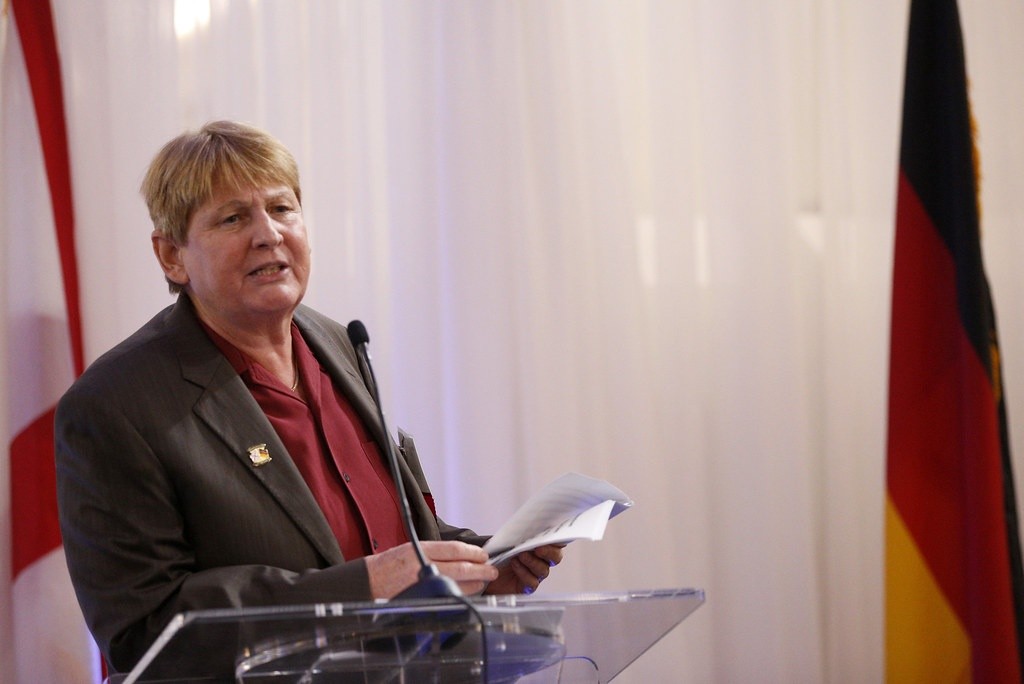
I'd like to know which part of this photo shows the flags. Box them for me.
[0,0,147,683]
[882,0,1024,683]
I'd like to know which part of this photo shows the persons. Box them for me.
[54,120,580,684]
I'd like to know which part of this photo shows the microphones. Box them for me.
[346,319,471,655]
